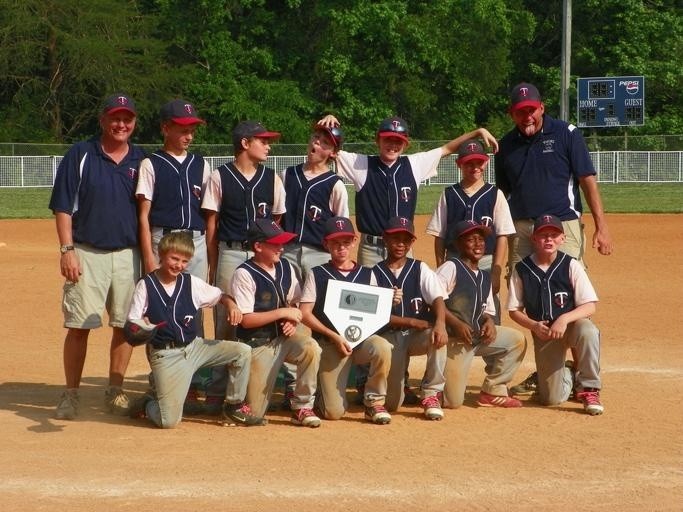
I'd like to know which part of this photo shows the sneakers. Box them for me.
[56,390,80,419]
[357,385,365,404]
[365,405,391,424]
[105,388,266,426]
[404,388,445,421]
[283,379,321,428]
[476,360,603,416]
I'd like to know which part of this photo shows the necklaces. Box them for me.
[331,259,357,272]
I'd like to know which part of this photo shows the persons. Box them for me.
[298,216,404,425]
[126,232,264,432]
[506,214,604,414]
[433,219,527,408]
[494,82,614,395]
[426,140,517,326]
[280,122,349,410]
[315,113,500,269]
[48,94,148,421]
[369,216,450,421]
[200,120,287,413]
[134,99,211,417]
[230,219,323,428]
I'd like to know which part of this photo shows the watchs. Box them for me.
[60,245,74,253]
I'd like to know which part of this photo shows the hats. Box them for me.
[458,140,490,164]
[313,123,342,148]
[232,121,280,145]
[535,214,564,235]
[511,82,541,110]
[384,216,417,240]
[103,95,136,118]
[325,217,358,239]
[246,219,297,244]
[379,118,410,145]
[453,220,493,240]
[160,99,205,125]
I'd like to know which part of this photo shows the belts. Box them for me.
[366,234,383,246]
[225,241,249,251]
[152,340,189,350]
[162,228,205,239]
